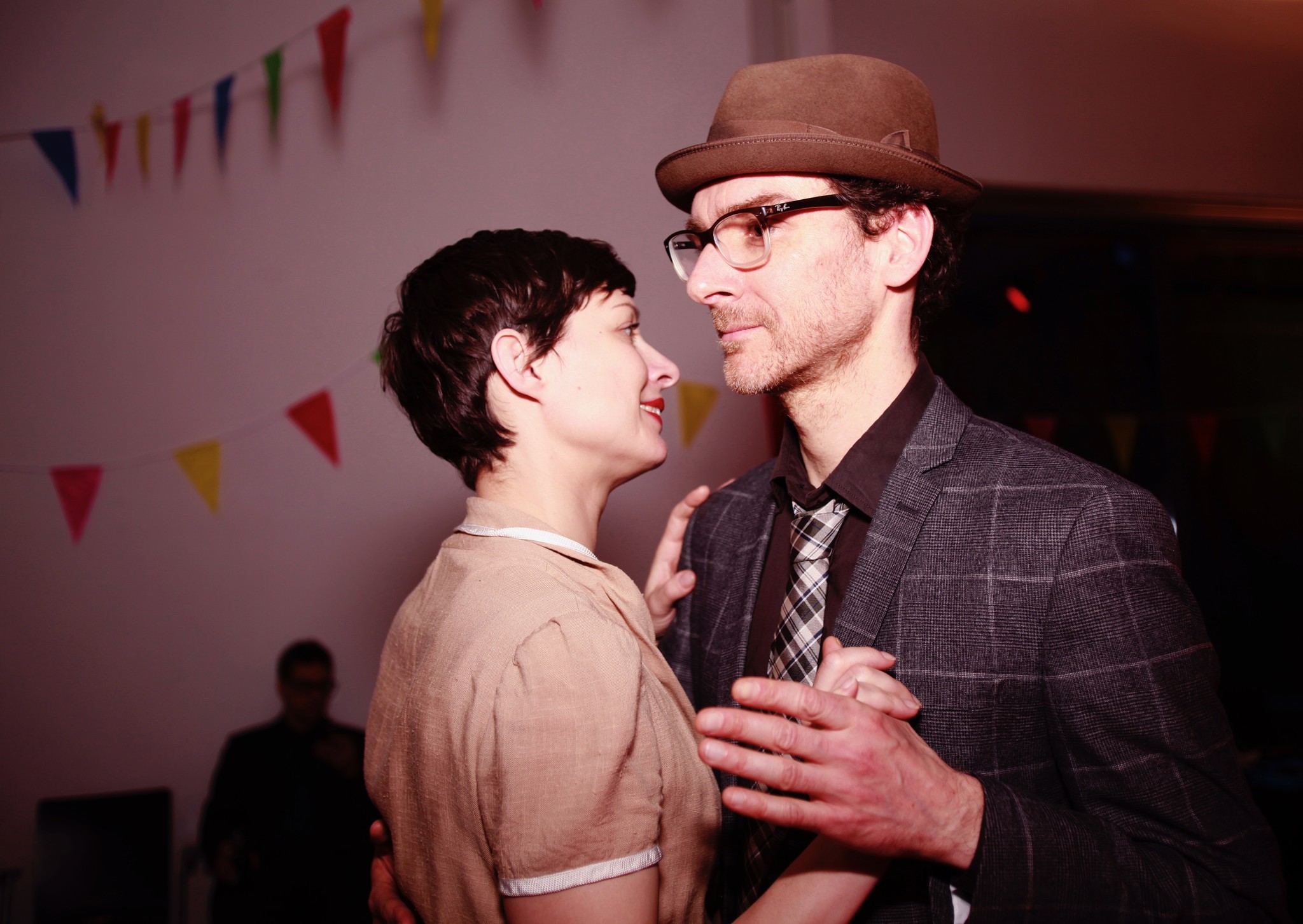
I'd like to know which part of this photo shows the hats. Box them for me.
[655,54,984,206]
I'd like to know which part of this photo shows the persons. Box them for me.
[197,639,381,924]
[366,54,1284,924]
[356,230,922,924]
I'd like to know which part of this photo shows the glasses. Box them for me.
[664,192,909,284]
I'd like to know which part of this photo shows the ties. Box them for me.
[755,496,849,793]
